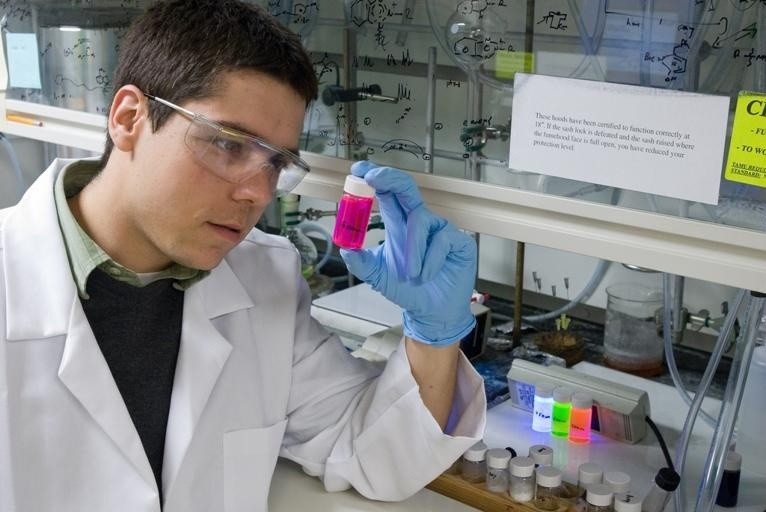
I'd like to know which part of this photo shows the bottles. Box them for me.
[552,388,572,436]
[531,384,554,433]
[717,453,742,508]
[331,174,377,250]
[461,443,681,512]
[569,390,594,445]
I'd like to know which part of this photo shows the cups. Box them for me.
[603,282,666,373]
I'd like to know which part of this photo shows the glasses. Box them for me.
[143,92,310,199]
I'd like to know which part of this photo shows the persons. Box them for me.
[0,0,490,512]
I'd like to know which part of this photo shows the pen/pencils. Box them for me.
[7,115,43,127]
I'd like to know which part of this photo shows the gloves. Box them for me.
[339,160,477,347]
